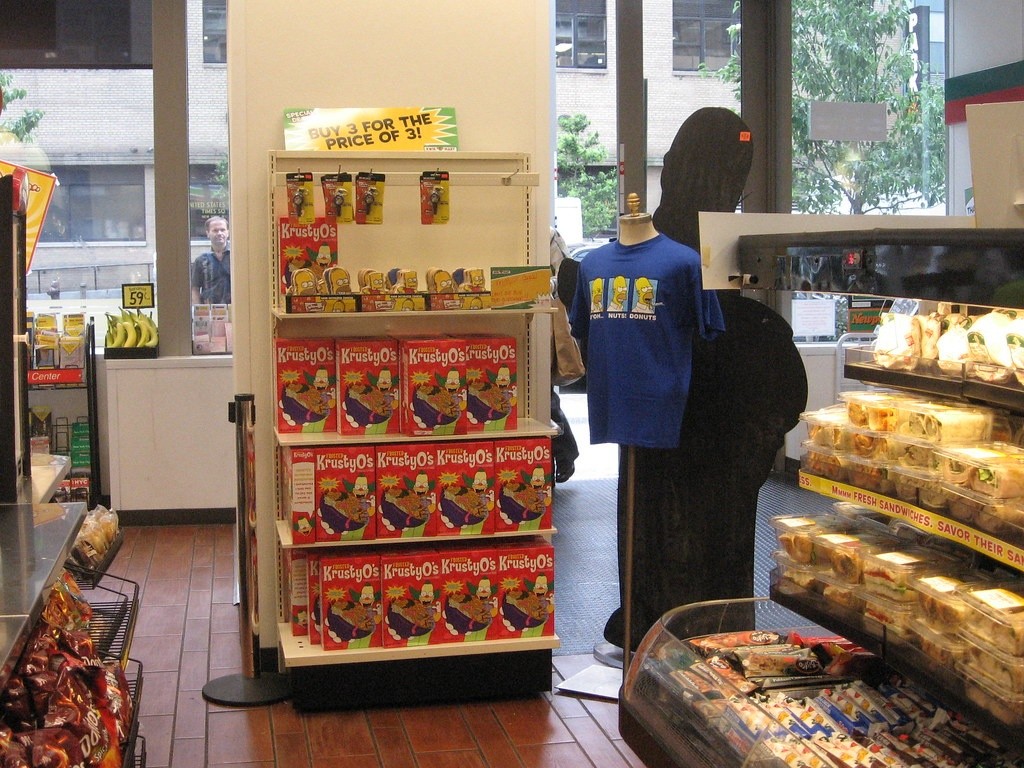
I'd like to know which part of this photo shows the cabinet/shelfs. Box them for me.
[270,149,557,714]
[0,169,144,768]
[619,210,1024,768]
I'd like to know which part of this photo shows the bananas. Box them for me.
[103,306,158,347]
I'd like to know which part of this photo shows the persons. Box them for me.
[568,213,726,449]
[549,226,579,483]
[191,215,231,306]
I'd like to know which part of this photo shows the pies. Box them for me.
[777,500,1024,718]
[802,394,1024,535]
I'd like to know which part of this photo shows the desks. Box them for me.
[106,356,237,525]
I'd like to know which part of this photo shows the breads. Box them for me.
[70,505,119,567]
[873,306,1024,386]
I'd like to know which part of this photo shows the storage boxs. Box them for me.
[275,333,519,435]
[103,330,159,358]
[282,441,553,652]
[772,388,1024,722]
